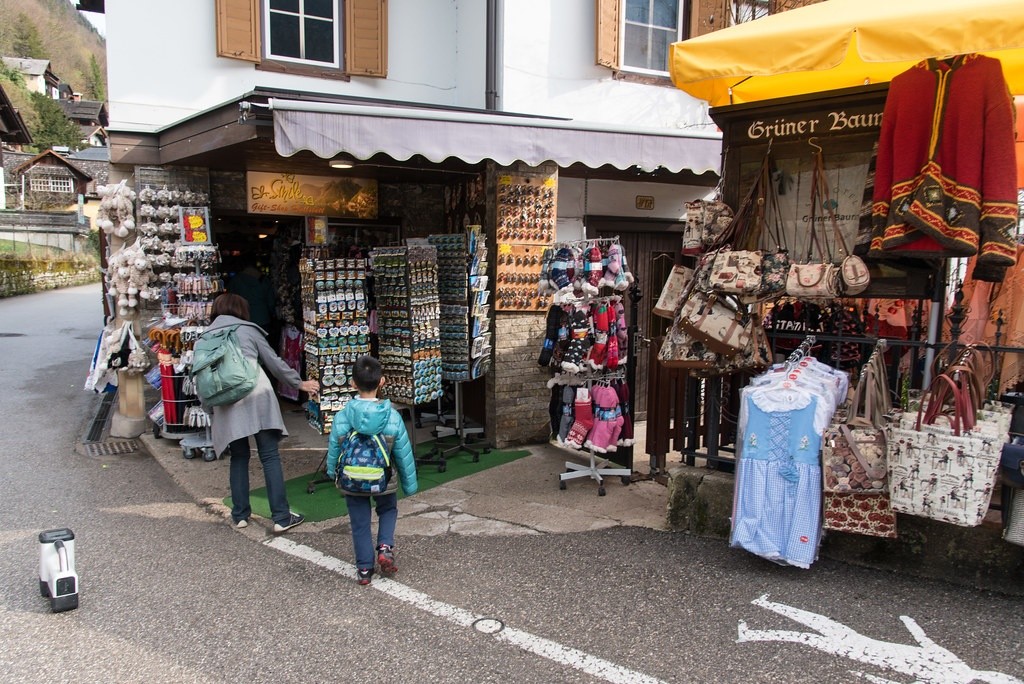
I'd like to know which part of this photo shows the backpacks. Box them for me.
[191,328,258,409]
[334,428,393,498]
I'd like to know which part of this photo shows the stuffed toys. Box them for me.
[94,182,136,238]
[106,241,154,315]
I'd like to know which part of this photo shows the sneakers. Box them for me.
[232,519,249,529]
[377,544,399,576]
[355,567,373,585]
[272,512,307,532]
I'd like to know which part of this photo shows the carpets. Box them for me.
[221,436,534,524]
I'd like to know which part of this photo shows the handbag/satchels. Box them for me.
[651,196,870,379]
[820,340,1023,544]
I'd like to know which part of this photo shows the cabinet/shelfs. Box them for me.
[539,236,635,497]
[373,245,450,476]
[171,244,221,457]
[431,233,493,462]
[294,256,374,495]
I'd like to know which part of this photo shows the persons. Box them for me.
[193,293,321,533]
[326,355,419,584]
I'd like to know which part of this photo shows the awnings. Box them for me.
[243,92,724,177]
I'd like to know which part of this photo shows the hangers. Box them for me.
[762,339,813,393]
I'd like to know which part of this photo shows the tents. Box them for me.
[666,0,1024,391]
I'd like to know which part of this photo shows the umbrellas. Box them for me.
[150,328,192,433]
